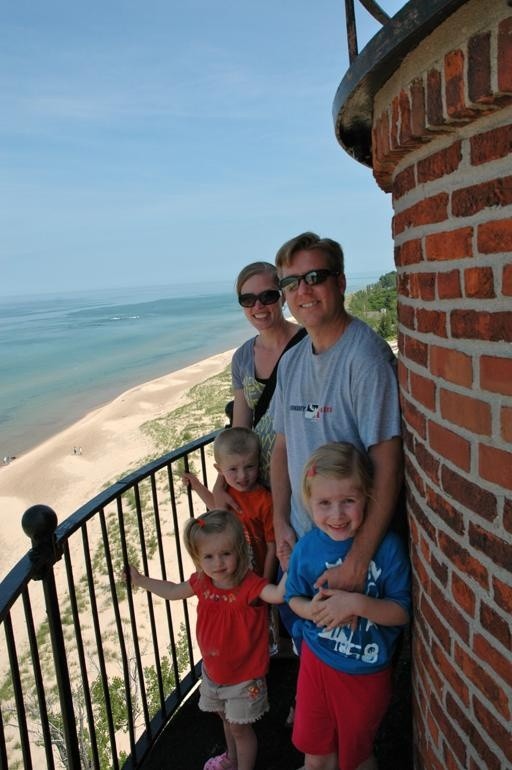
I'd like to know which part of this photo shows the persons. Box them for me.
[179,423,283,668]
[267,229,406,729]
[210,257,301,518]
[277,438,412,770]
[115,504,287,769]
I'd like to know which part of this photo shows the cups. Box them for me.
[239,289,283,308]
[278,269,336,293]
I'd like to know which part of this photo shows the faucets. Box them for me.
[203,751,237,770]
